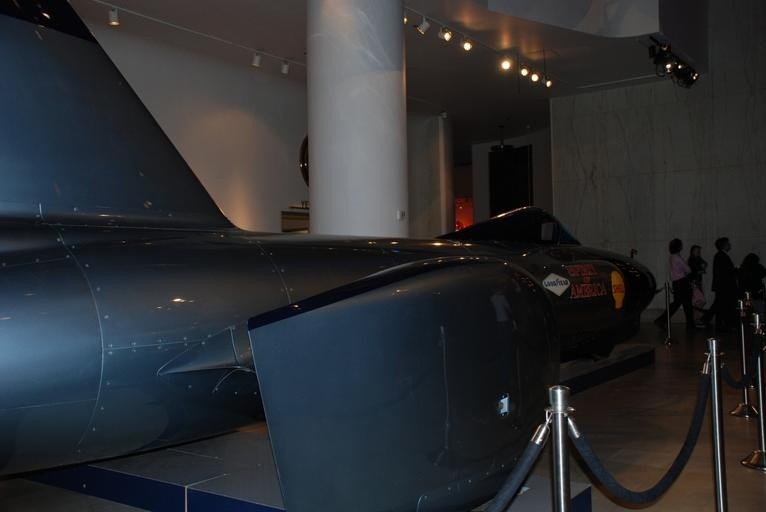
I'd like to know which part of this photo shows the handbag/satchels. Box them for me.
[692,285,706,308]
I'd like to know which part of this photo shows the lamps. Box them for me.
[401,10,476,54]
[650,35,701,89]
[249,53,292,77]
[107,7,122,26]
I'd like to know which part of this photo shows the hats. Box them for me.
[715,236,729,247]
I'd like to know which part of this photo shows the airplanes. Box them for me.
[0,0,659,512]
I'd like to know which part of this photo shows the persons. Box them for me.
[655,236,736,327]
